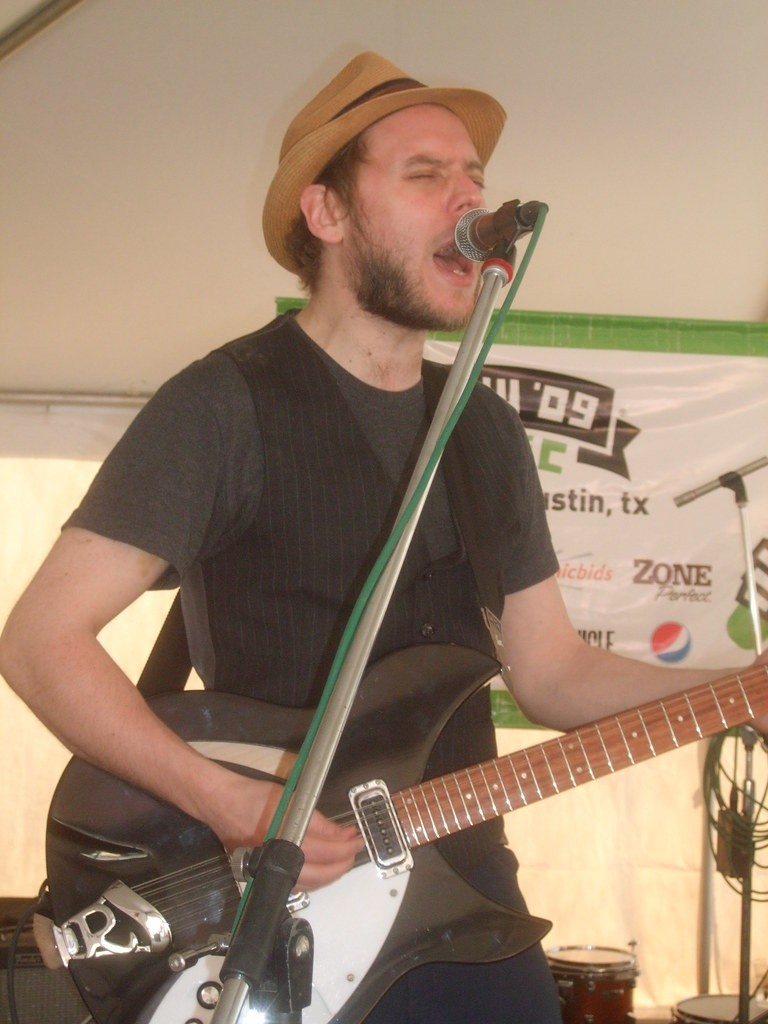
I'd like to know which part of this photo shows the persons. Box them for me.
[0,50,768,1024]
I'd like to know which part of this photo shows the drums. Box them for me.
[667,993,767,1024]
[545,940,641,1024]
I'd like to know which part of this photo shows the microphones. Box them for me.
[454,200,549,262]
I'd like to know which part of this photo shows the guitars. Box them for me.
[35,656,768,1024]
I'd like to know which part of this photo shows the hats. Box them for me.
[263,51,508,275]
